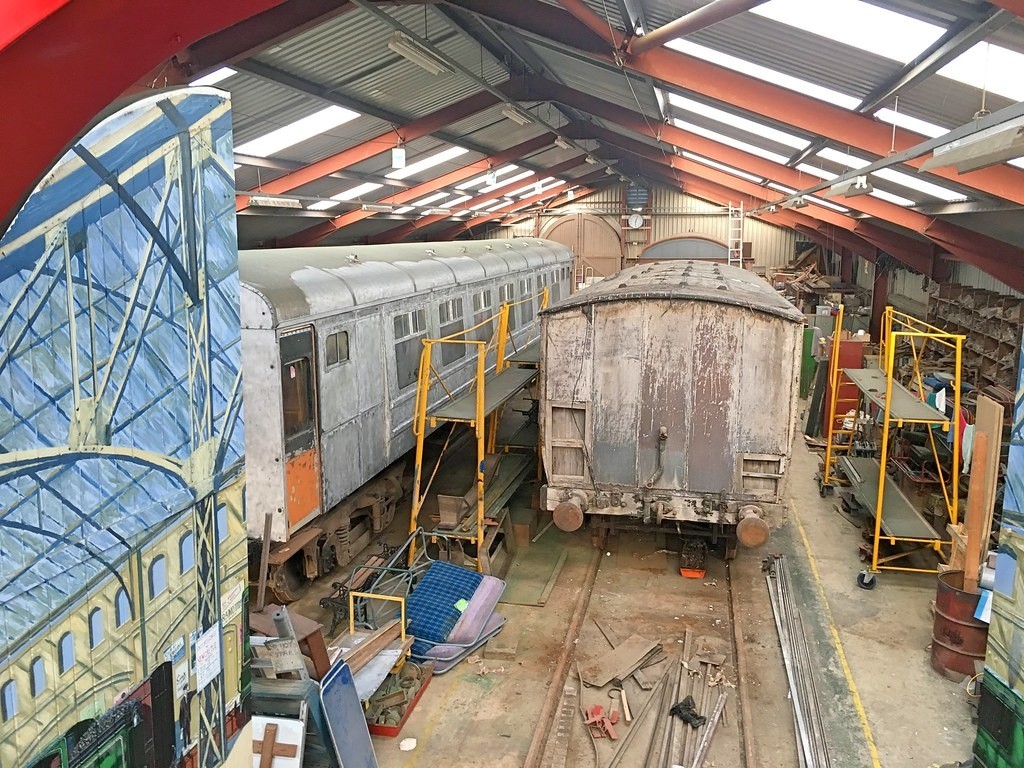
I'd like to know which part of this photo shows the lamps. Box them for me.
[388,5,624,182]
[769,120,1023,213]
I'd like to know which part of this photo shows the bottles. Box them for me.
[818,337,827,358]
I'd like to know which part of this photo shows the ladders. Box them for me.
[728,200,744,270]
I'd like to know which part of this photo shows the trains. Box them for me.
[235,236,575,606]
[538,260,807,559]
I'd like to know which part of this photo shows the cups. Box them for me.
[858,329,864,335]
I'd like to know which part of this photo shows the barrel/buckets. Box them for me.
[931,570,989,683]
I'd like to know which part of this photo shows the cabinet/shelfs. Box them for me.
[925,281,1024,395]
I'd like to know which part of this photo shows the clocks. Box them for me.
[628,214,643,228]
